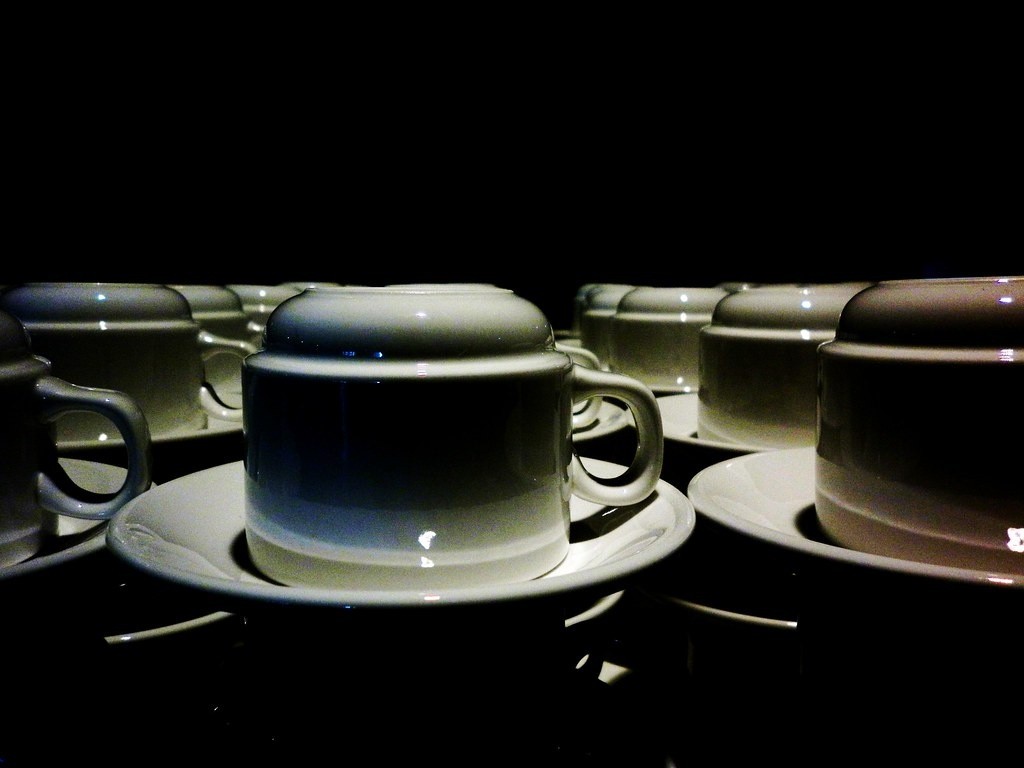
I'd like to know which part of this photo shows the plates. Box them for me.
[688,447,1024,585]
[572,399,629,441]
[56,393,243,453]
[106,455,696,630]
[0,458,157,577]
[626,393,776,451]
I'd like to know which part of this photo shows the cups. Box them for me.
[610,287,726,391]
[0,311,151,570]
[229,285,299,350]
[571,285,597,336]
[0,283,258,441]
[815,276,1024,574]
[582,284,636,372]
[167,286,265,398]
[697,284,871,448]
[243,288,664,591]
